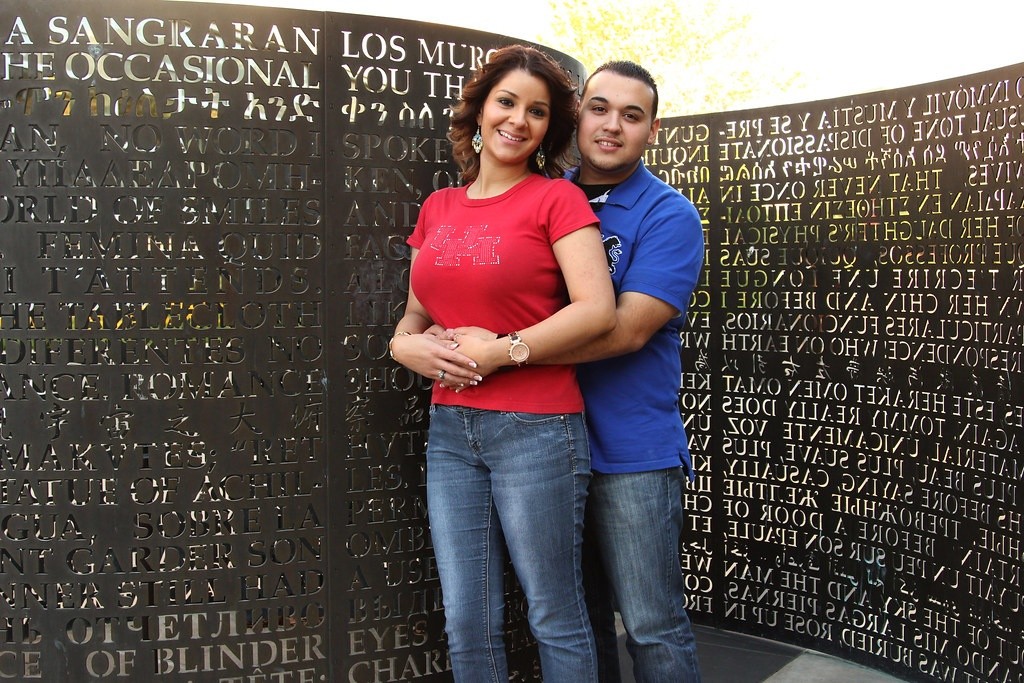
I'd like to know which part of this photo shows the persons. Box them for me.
[392,45,617,683]
[422,59,704,683]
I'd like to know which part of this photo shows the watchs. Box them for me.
[507,330,530,367]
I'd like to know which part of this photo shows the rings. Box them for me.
[439,370,446,379]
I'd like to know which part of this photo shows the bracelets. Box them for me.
[389,331,411,361]
